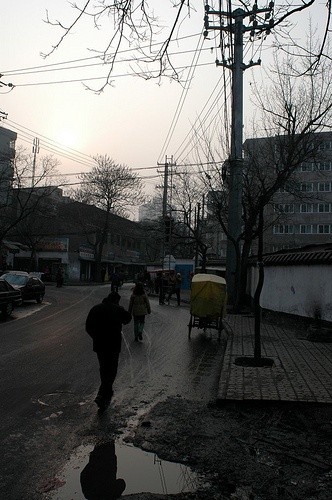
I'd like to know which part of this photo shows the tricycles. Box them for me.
[187,274,228,341]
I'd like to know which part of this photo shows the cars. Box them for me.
[0,271,45,307]
[0,278,22,319]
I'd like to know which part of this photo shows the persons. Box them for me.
[128,282,151,341]
[56,268,64,287]
[109,271,194,306]
[80,440,126,500]
[43,266,50,282]
[85,292,132,409]
